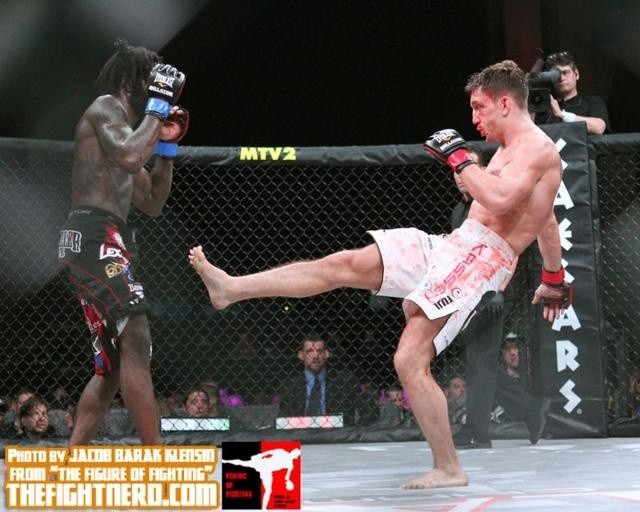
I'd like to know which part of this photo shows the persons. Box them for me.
[57,38,192,448]
[524,50,612,136]
[449,147,551,450]
[188,60,574,491]
[607,362,640,420]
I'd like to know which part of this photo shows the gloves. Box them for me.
[425,128,478,174]
[146,64,185,121]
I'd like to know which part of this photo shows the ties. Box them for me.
[307,378,322,416]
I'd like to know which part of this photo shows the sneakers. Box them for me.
[524,394,551,444]
[451,423,493,450]
